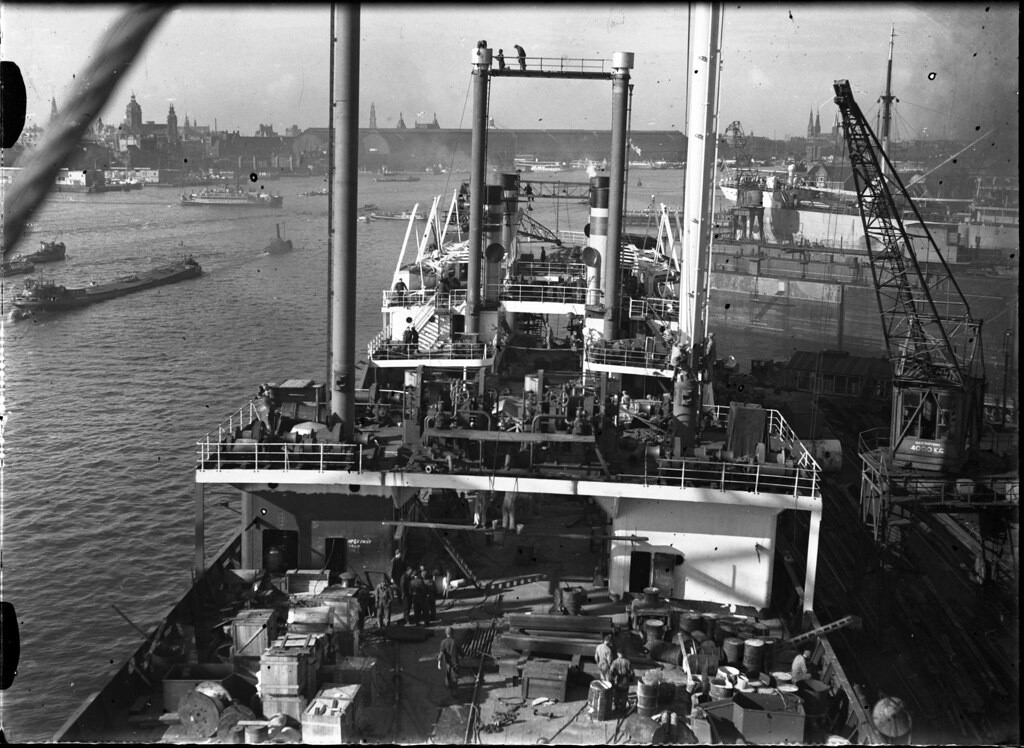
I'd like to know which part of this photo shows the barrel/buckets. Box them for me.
[698,648,727,665]
[773,672,799,693]
[721,621,778,670]
[636,680,659,715]
[649,641,684,666]
[674,630,711,647]
[709,678,731,699]
[686,655,719,676]
[588,680,612,722]
[560,588,581,614]
[244,725,268,745]
[644,620,664,641]
[626,714,665,745]
[680,611,719,630]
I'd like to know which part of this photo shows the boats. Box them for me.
[370,210,427,220]
[759,77,1022,747]
[51,4,915,747]
[310,188,328,195]
[23,241,66,263]
[297,191,309,197]
[9,254,202,311]
[672,28,1024,305]
[0,253,35,276]
[622,195,728,240]
[372,175,420,183]
[55,166,143,193]
[514,153,667,172]
[263,222,292,255]
[180,170,283,206]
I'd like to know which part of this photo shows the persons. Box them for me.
[622,391,630,408]
[541,247,545,266]
[375,549,435,628]
[395,278,408,305]
[437,626,463,693]
[403,326,420,353]
[595,635,635,713]
[576,275,586,302]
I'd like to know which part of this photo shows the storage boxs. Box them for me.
[160,567,380,745]
[731,693,807,743]
[522,660,569,702]
